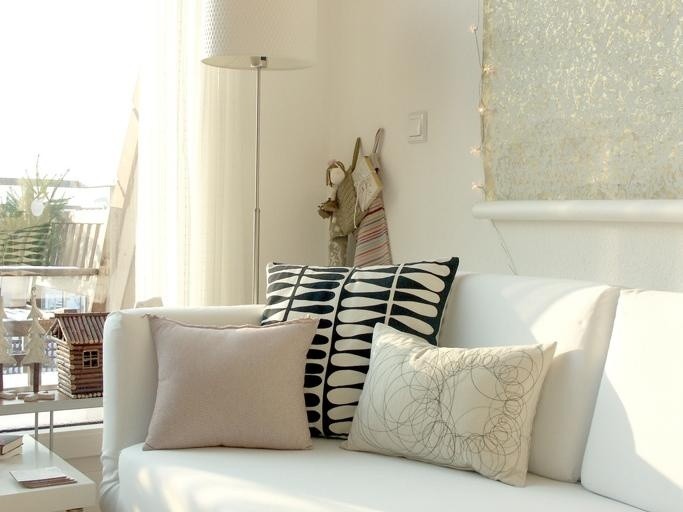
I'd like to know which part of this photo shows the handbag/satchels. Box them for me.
[329,169,366,239]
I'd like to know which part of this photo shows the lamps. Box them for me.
[195,0,319,308]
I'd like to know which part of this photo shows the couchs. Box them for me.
[99,270,683,512]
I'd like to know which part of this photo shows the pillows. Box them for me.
[259,255,462,444]
[140,311,322,452]
[341,321,556,489]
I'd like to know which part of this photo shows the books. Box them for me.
[0,434,24,455]
[9,467,77,488]
